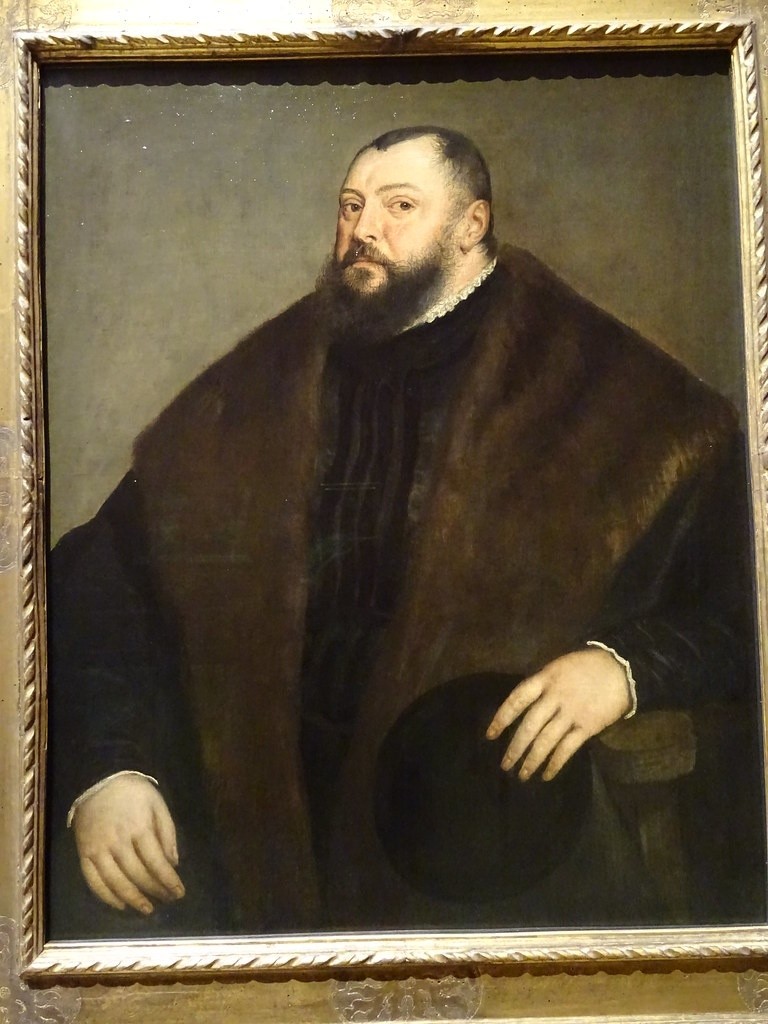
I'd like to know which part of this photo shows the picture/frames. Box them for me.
[0,0,768,982]
